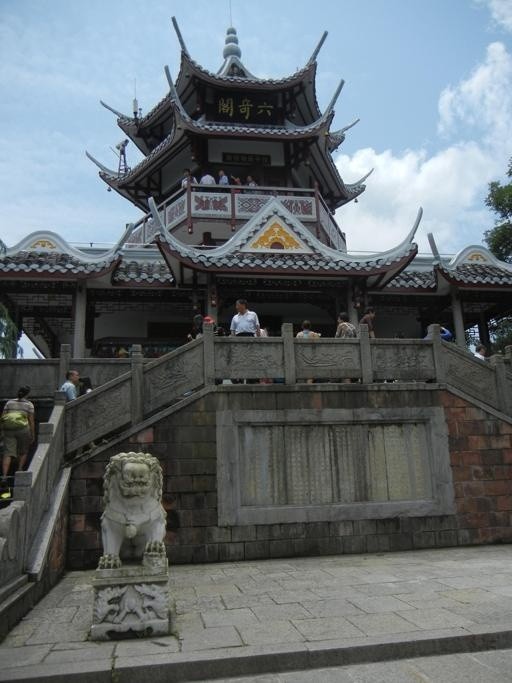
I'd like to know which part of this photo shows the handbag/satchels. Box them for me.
[1,412,29,431]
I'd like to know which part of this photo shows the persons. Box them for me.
[60,369,84,462]
[243,174,261,195]
[392,326,407,338]
[78,376,92,395]
[3,386,36,482]
[200,170,216,185]
[180,168,198,192]
[218,168,229,185]
[473,344,486,360]
[424,325,452,339]
[230,174,241,193]
[188,296,374,384]
